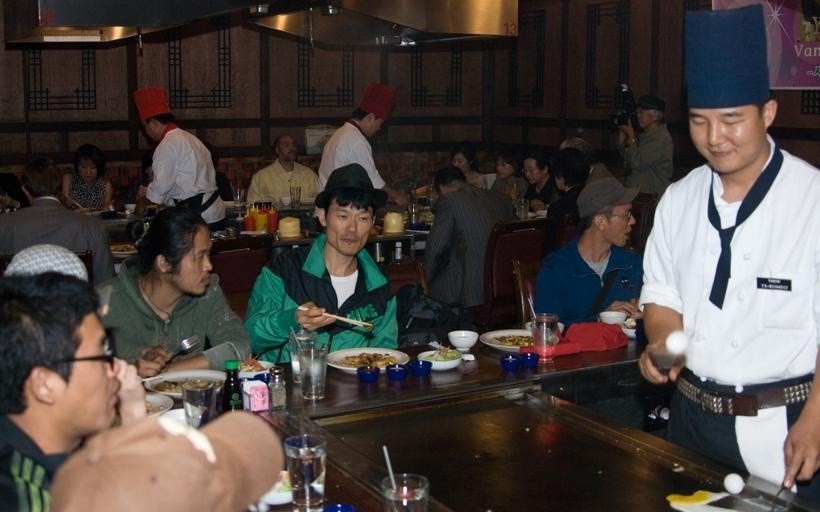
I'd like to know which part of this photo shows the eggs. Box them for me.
[724,473,745,495]
[665,329,689,354]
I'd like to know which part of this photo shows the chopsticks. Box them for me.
[297,305,373,330]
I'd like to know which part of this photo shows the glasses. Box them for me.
[54,348,114,371]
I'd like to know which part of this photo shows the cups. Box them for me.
[408,178,440,224]
[298,341,328,398]
[500,353,538,368]
[357,361,433,383]
[285,435,328,510]
[233,189,244,210]
[529,312,557,361]
[517,199,530,220]
[380,472,431,511]
[289,331,314,384]
[291,185,301,206]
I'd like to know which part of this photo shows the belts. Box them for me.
[676,376,812,416]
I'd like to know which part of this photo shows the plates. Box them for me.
[140,360,274,427]
[517,212,539,218]
[419,351,462,370]
[324,346,412,372]
[109,244,139,258]
[476,328,559,351]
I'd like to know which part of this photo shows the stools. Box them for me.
[377,258,427,318]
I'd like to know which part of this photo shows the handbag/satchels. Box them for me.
[395,284,466,347]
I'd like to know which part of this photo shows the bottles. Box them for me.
[236,203,279,234]
[650,327,689,376]
[268,365,288,409]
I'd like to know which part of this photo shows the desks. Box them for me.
[0,167,431,268]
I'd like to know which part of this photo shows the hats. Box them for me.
[134,86,171,124]
[359,81,397,122]
[684,4,771,108]
[635,96,666,111]
[576,162,640,218]
[315,163,388,208]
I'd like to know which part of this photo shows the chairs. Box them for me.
[203,232,274,322]
[627,190,661,252]
[469,214,559,330]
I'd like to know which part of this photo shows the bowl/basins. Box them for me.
[281,198,291,205]
[526,321,565,335]
[124,204,137,214]
[599,311,627,325]
[448,329,482,351]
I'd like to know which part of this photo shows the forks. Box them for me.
[166,333,201,363]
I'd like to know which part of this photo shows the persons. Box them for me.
[633,98,820,493]
[241,160,404,363]
[92,206,252,379]
[0,270,146,512]
[533,174,647,337]
[1,77,678,314]
